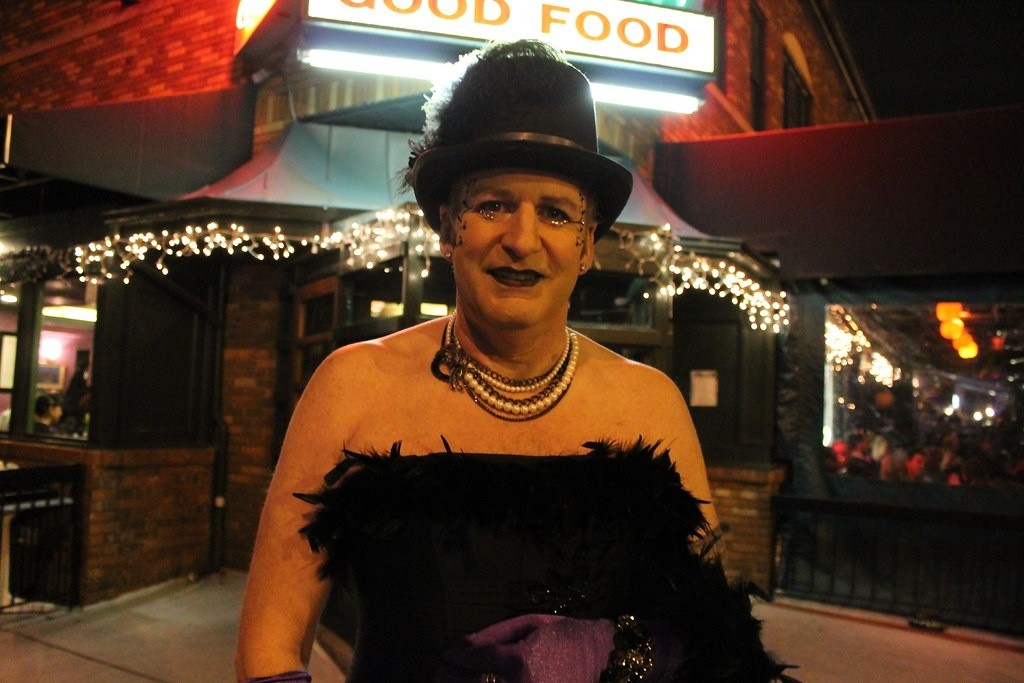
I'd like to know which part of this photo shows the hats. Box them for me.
[394,40,632,243]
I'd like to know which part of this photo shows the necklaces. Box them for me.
[431,308,580,422]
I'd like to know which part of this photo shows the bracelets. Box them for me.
[238,671,312,683]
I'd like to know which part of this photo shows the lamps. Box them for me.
[294,44,708,117]
[936,302,978,358]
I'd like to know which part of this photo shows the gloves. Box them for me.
[419,614,617,683]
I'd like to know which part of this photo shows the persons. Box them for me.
[823,408,1024,492]
[235,46,802,683]
[33,360,93,437]
